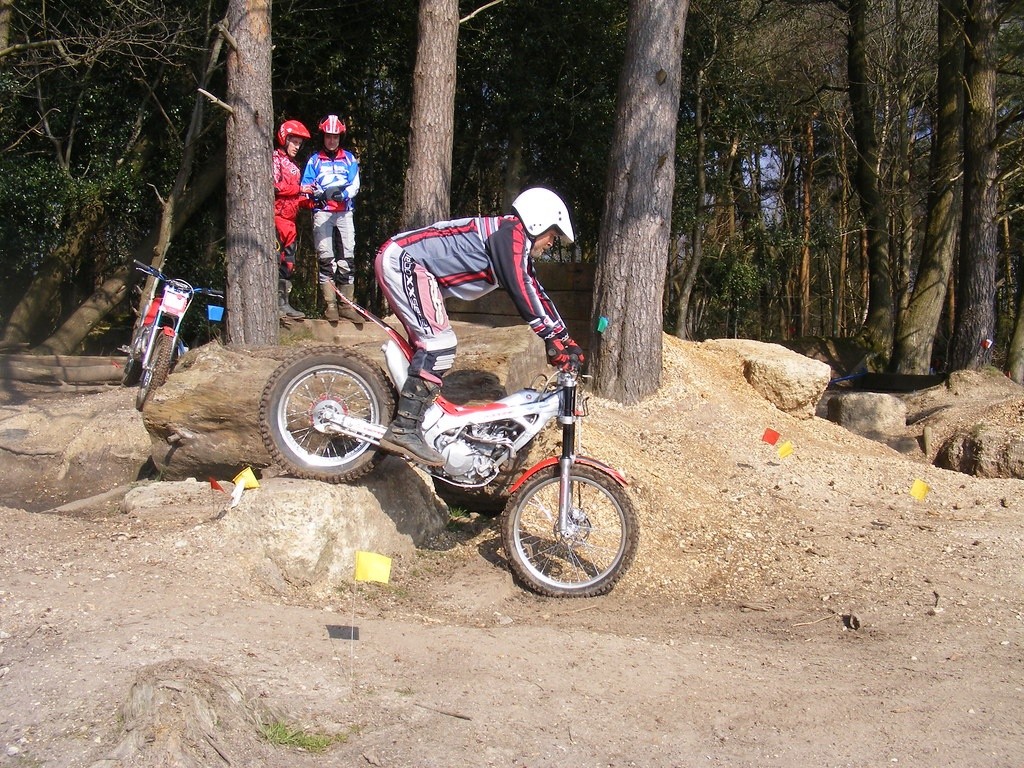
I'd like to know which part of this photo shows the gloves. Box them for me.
[332,191,343,202]
[301,185,314,193]
[544,333,584,371]
[315,200,327,209]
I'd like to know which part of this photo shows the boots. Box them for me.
[380,375,447,466]
[277,279,305,319]
[321,282,365,323]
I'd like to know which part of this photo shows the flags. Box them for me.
[1006,369,1011,378]
[209,476,227,493]
[178,340,186,358]
[911,479,929,500]
[980,338,993,349]
[233,468,261,489]
[111,359,119,367]
[789,324,798,333]
[762,427,779,445]
[778,439,794,459]
[207,305,225,321]
[231,478,245,508]
[597,316,608,332]
[354,550,391,584]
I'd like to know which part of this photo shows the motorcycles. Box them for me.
[258,273,640,597]
[117,257,227,410]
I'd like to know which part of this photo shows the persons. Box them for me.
[273,120,328,322]
[375,187,585,466]
[301,114,365,323]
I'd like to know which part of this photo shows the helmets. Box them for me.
[276,120,311,146]
[318,115,346,135]
[512,188,574,244]
